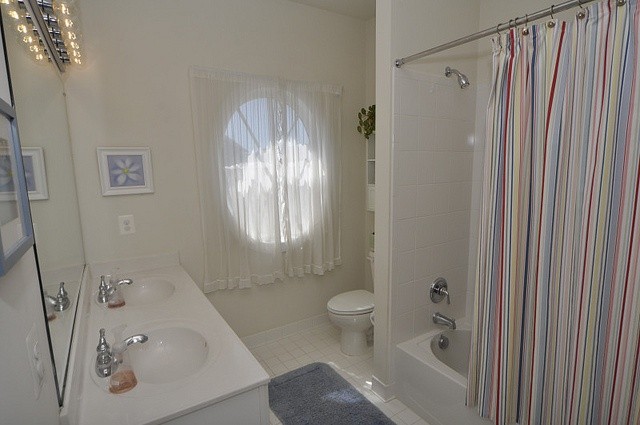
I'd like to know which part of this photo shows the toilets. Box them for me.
[327,246,373,355]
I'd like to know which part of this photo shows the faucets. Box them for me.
[432,313,458,334]
[97,271,133,306]
[45,293,61,306]
[97,334,147,362]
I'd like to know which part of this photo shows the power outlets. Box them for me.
[117,214,135,235]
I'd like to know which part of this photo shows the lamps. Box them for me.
[2,1,53,66]
[29,0,82,73]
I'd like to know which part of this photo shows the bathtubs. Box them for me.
[393,316,494,424]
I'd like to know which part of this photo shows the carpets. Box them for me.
[268,362,396,425]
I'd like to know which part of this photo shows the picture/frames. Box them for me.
[95,145,154,196]
[1,147,49,201]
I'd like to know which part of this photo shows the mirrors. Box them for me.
[1,1,87,407]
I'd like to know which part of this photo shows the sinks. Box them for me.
[118,279,176,307]
[118,326,210,385]
